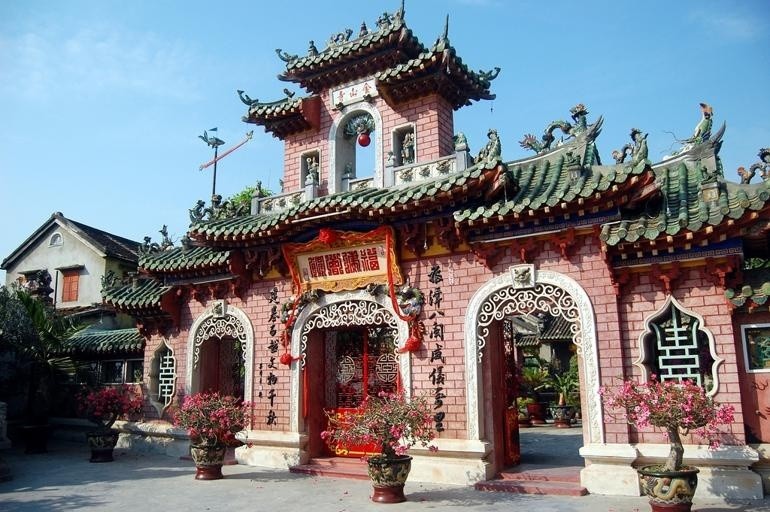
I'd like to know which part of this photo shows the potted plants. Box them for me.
[523,345,581,428]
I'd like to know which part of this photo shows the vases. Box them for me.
[189,443,225,478]
[84,431,120,462]
[525,402,549,424]
[636,464,701,510]
[366,453,413,502]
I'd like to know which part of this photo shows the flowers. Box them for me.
[166,390,256,447]
[596,373,743,468]
[76,382,145,433]
[320,390,439,457]
